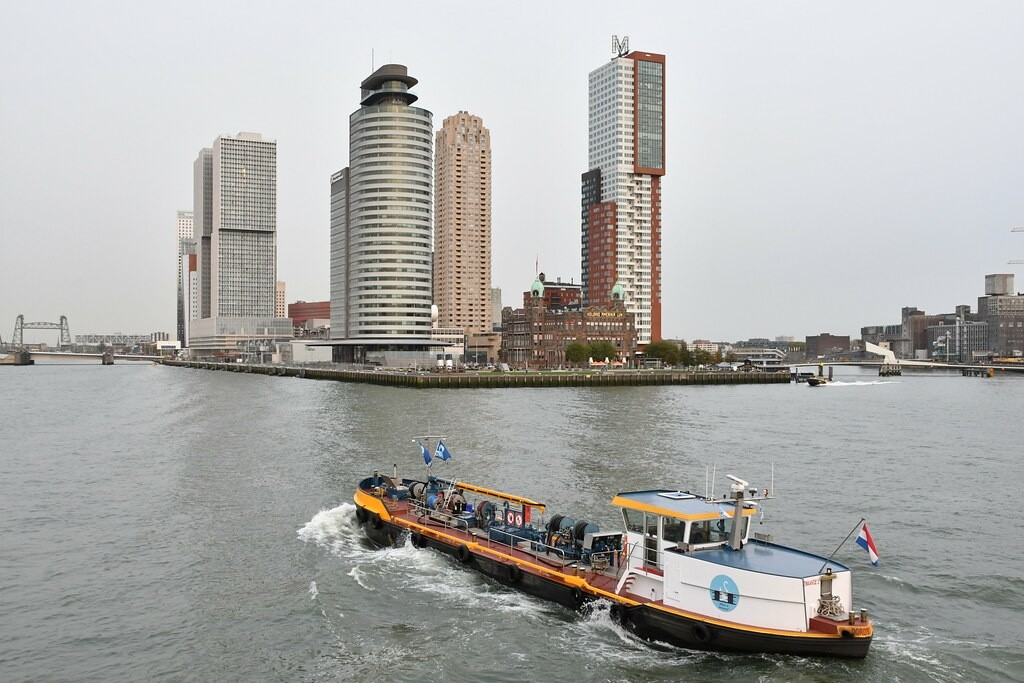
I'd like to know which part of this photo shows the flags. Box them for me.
[854,522,879,567]
[435,440,452,462]
[419,442,432,465]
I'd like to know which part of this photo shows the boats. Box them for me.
[806,377,829,386]
[351,416,874,665]
[879,351,901,375]
